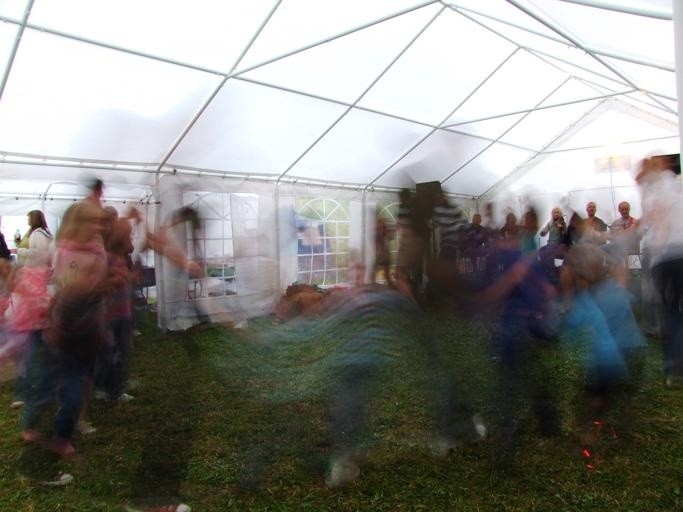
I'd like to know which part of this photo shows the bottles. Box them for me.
[14,229,20,242]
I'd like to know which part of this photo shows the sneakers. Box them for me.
[73,419,97,435]
[94,390,136,403]
[25,469,74,488]
[124,498,193,512]
[9,398,25,410]
[47,439,77,457]
[18,429,44,443]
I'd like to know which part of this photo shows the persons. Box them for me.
[207,277,403,488]
[0,173,148,487]
[125,176,220,511]
[376,150,683,473]
